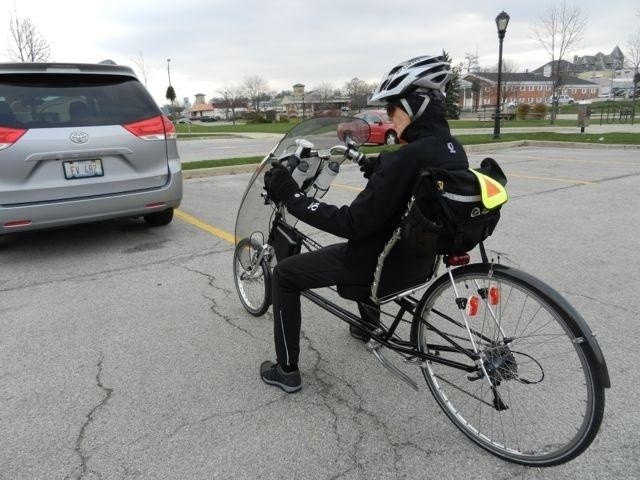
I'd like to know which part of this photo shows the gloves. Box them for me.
[360,156,380,180]
[263,160,306,208]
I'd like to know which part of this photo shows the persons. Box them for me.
[258,51,470,395]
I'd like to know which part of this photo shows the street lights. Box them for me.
[493,9,510,138]
[302,94,305,121]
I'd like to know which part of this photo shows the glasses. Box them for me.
[386,103,401,116]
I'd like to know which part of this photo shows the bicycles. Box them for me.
[232,114,611,468]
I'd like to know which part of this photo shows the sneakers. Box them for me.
[261,360,302,392]
[349,318,386,347]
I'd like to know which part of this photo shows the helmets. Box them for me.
[367,56,456,105]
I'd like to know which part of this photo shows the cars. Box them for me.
[179,116,217,124]
[336,108,407,144]
[507,99,519,109]
[0,58,183,235]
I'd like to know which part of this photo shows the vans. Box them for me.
[550,94,574,105]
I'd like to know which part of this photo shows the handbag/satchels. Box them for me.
[400,158,507,255]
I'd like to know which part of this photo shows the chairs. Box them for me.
[69,100,89,119]
[337,173,441,306]
